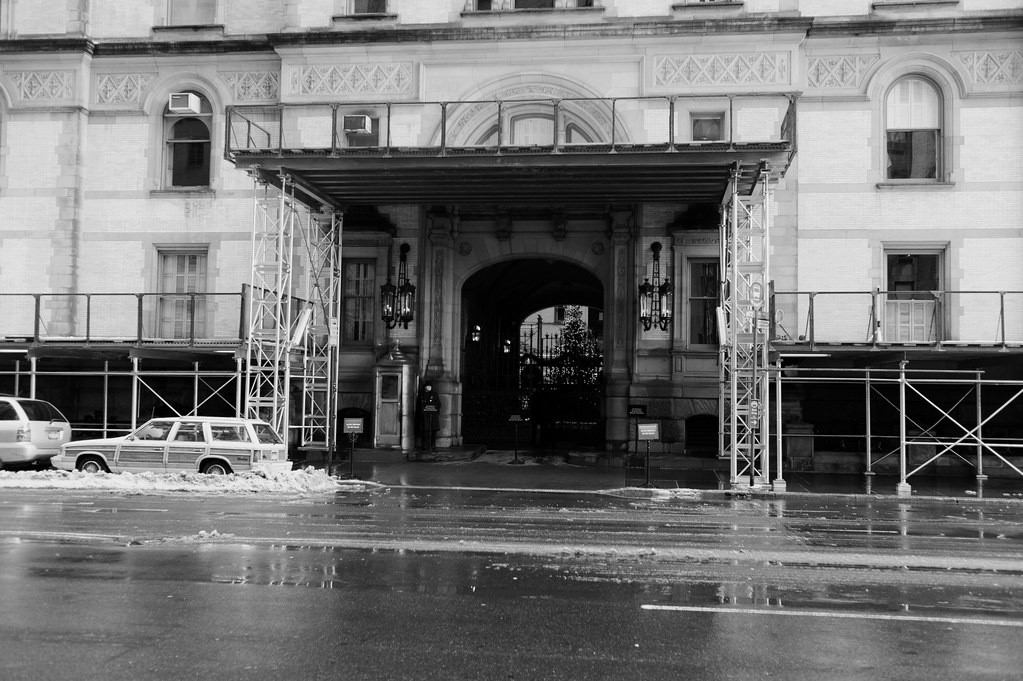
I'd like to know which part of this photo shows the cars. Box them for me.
[0,391,74,471]
[50,415,294,477]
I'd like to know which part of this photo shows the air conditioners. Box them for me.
[342,114,372,134]
[169,93,201,113]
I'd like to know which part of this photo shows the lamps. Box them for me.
[638,241,676,332]
[380,242,416,330]
